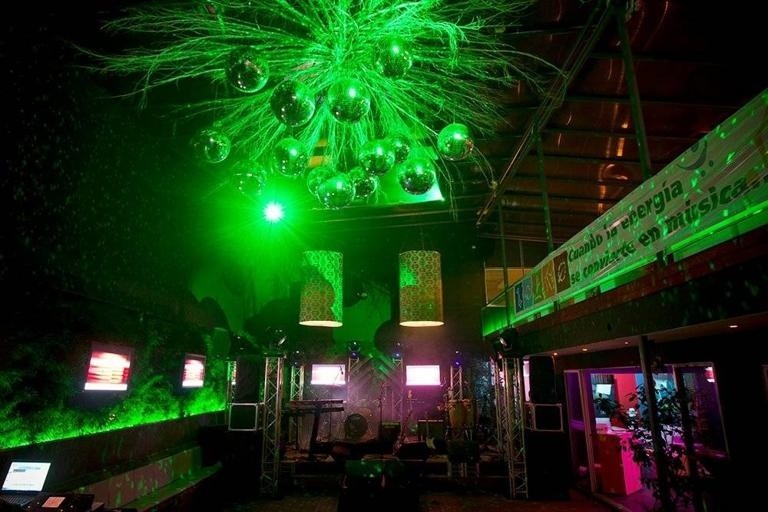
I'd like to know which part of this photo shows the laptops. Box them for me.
[0,459,52,507]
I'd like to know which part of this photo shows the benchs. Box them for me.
[49,440,222,512]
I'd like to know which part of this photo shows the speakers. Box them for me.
[445,438,479,465]
[398,442,430,463]
[234,354,263,403]
[529,355,558,404]
[378,421,400,453]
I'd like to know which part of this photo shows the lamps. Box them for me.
[299,250,343,327]
[396,225,445,328]
